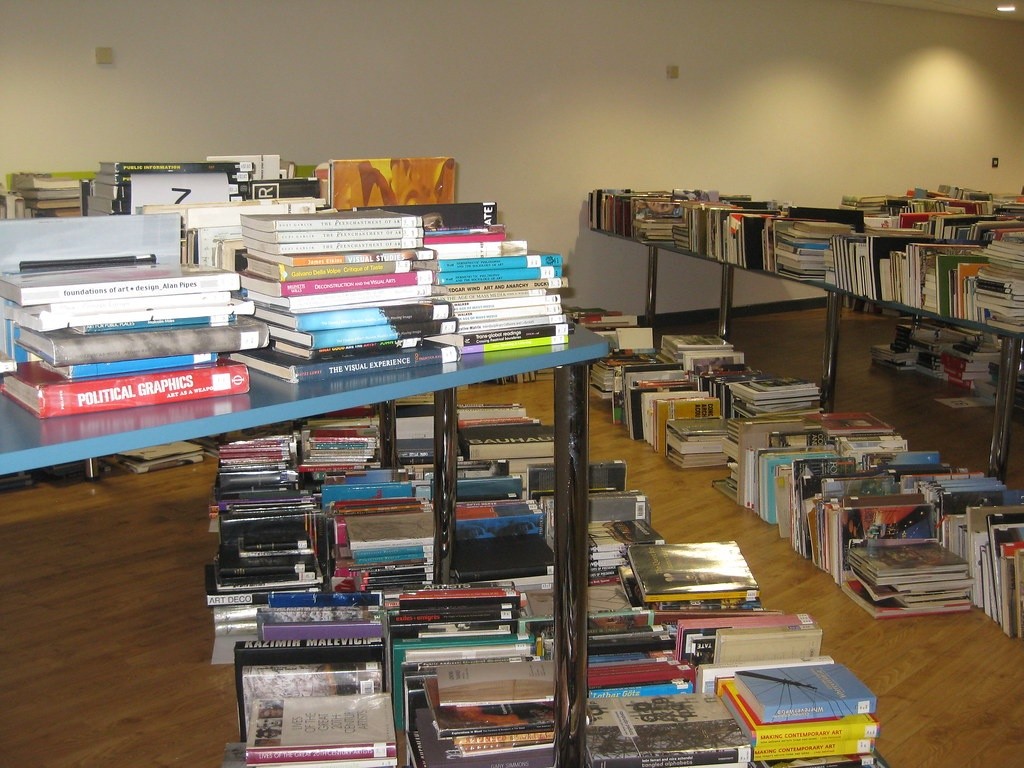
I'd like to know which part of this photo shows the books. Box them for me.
[588,184,1024,336]
[204,404,893,768]
[544,306,1024,639]
[0,154,574,474]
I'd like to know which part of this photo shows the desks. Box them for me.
[0,320,609,768]
[589,227,1024,484]
